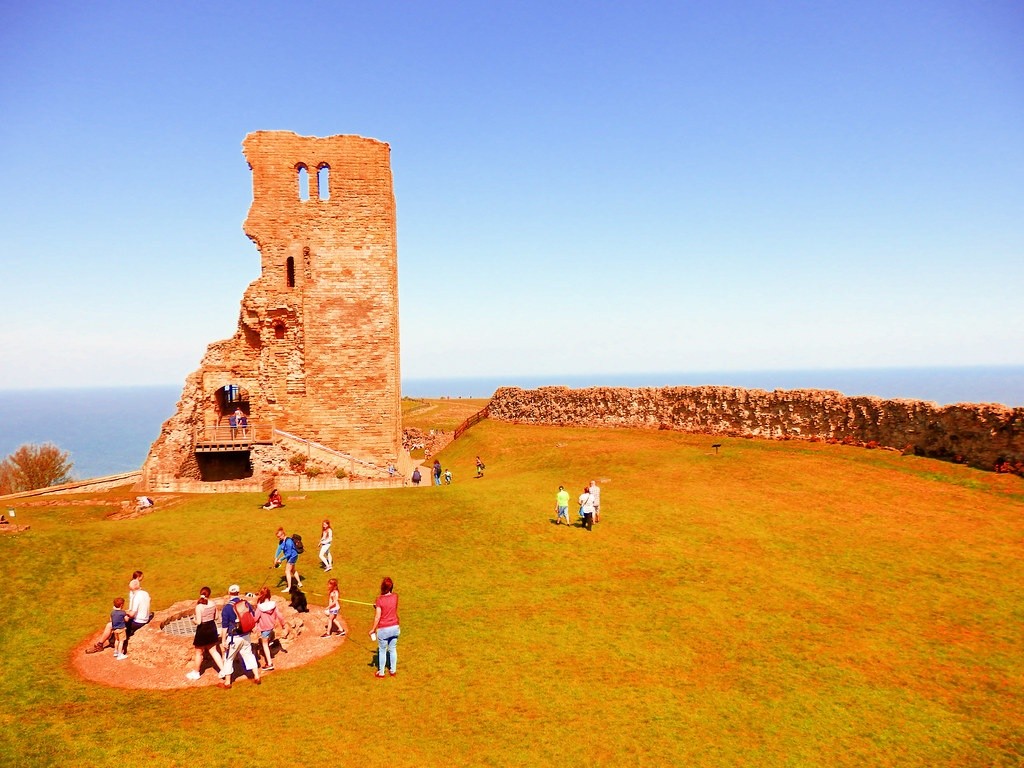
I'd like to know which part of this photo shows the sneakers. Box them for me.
[262,663,274,670]
[375,671,385,678]
[298,583,303,587]
[253,677,261,684]
[186,670,200,679]
[85,642,104,654]
[324,564,332,572]
[388,670,396,677]
[113,652,117,656]
[335,629,346,636]
[321,633,332,638]
[217,682,232,689]
[281,588,290,592]
[219,667,233,678]
[117,654,128,660]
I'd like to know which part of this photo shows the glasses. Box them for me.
[277,535,282,538]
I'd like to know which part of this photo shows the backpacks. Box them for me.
[285,534,304,554]
[436,464,441,474]
[480,461,485,470]
[414,471,420,481]
[227,599,255,633]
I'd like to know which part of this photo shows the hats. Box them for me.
[229,585,240,592]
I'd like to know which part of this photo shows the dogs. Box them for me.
[277,575,305,587]
[288,585,310,613]
[244,588,271,604]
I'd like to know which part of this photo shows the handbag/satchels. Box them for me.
[579,506,584,516]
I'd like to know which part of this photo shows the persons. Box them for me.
[386,461,395,478]
[272,527,304,593]
[368,577,400,678]
[317,519,333,573]
[432,459,452,487]
[320,578,346,639]
[85,571,152,661]
[229,407,248,441]
[411,466,422,487]
[578,480,601,531]
[262,489,281,510]
[554,485,570,526]
[475,455,482,476]
[184,584,285,689]
